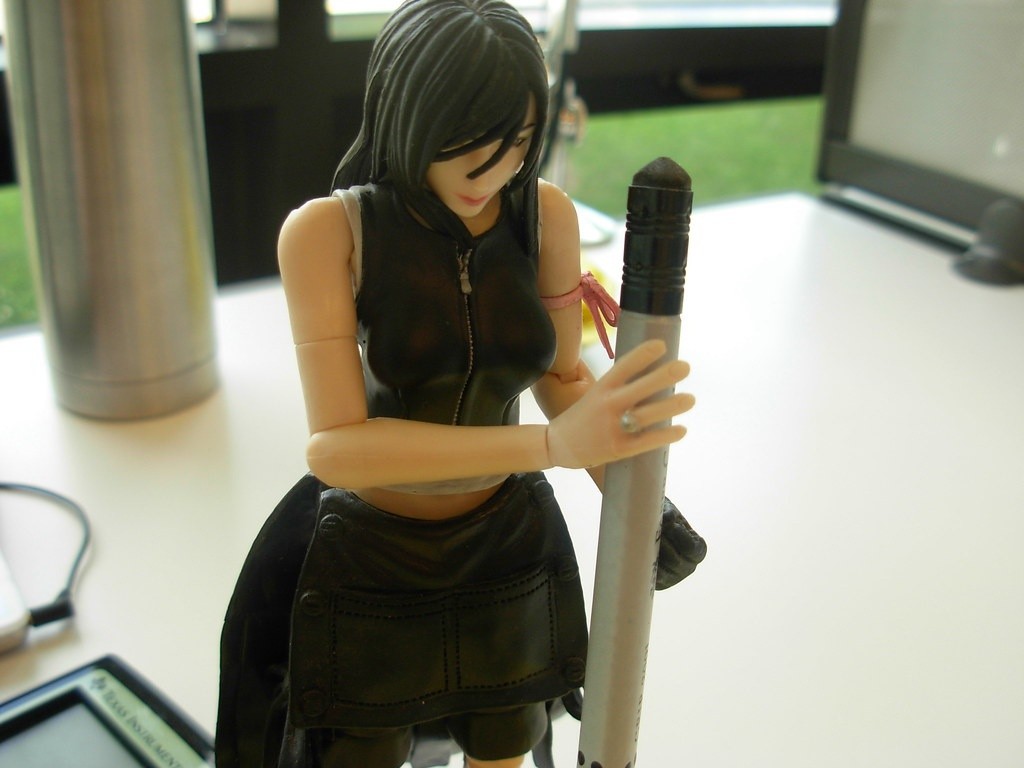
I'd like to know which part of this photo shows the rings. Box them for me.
[621,409,642,434]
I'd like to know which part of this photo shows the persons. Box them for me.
[211,0,706,768]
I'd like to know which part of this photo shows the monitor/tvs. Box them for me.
[814,0,1024,265]
